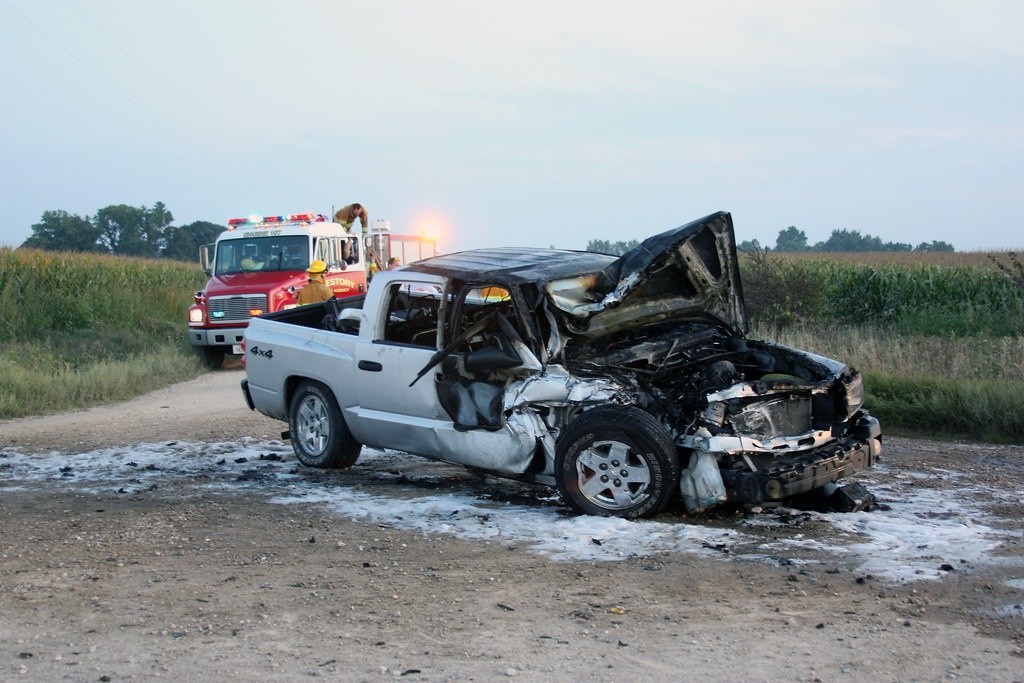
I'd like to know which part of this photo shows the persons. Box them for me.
[333,203,369,236]
[388,257,401,271]
[296,259,334,308]
[341,237,365,265]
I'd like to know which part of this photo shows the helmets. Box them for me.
[240,259,255,271]
[306,261,328,274]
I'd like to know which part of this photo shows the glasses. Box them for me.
[389,257,401,263]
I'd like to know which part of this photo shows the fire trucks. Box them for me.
[187,206,438,371]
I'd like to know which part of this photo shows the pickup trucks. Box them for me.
[240,211,884,522]
